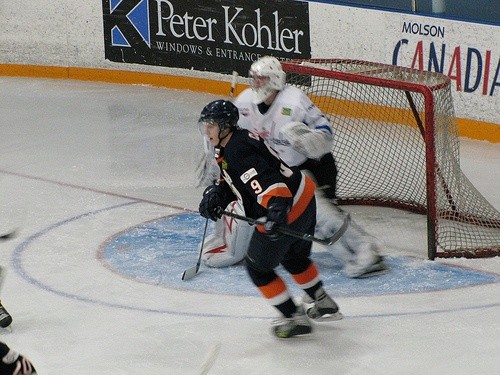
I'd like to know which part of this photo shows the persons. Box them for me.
[0,303,39,375]
[199,99,344,341]
[201,56,383,278]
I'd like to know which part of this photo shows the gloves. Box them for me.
[264,196,288,243]
[199,184,233,221]
[281,122,334,161]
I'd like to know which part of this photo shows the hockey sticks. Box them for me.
[181,70,240,282]
[219,209,354,245]
[392,38,459,210]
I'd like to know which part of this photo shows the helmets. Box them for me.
[247,55,287,104]
[197,99,240,130]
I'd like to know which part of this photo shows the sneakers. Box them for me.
[267,303,318,341]
[0,297,13,334]
[305,286,345,323]
